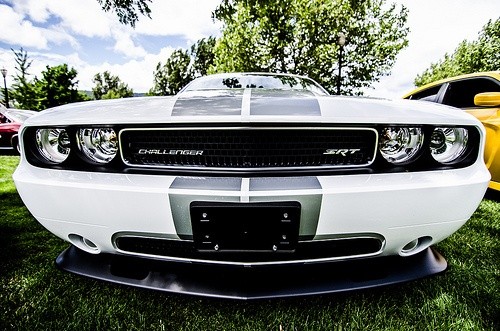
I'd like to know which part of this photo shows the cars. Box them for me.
[398,72,500,192]
[0,102,36,153]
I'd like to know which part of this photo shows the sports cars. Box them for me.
[12,72,494,302]
